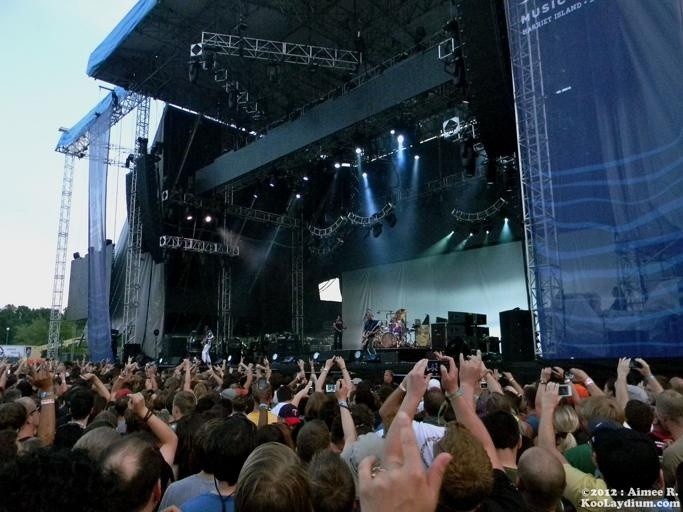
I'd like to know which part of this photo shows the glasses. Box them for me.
[27,403,40,416]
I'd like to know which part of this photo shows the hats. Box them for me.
[586,417,623,442]
[278,403,300,424]
[426,377,441,391]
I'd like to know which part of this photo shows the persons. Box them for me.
[361,312,383,359]
[332,313,347,349]
[199,324,214,364]
[609,286,626,310]
[0,352,683,512]
[390,308,407,333]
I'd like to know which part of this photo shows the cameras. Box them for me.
[558,385,573,398]
[498,373,505,378]
[480,382,488,390]
[424,360,450,378]
[563,371,574,380]
[629,360,640,368]
[324,382,342,393]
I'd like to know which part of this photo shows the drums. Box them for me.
[381,332,398,348]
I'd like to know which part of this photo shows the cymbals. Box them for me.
[396,309,407,314]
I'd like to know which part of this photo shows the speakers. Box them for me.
[431,311,500,355]
[499,309,535,361]
[126,157,161,253]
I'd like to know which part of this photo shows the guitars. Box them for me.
[361,321,382,344]
[201,332,212,345]
